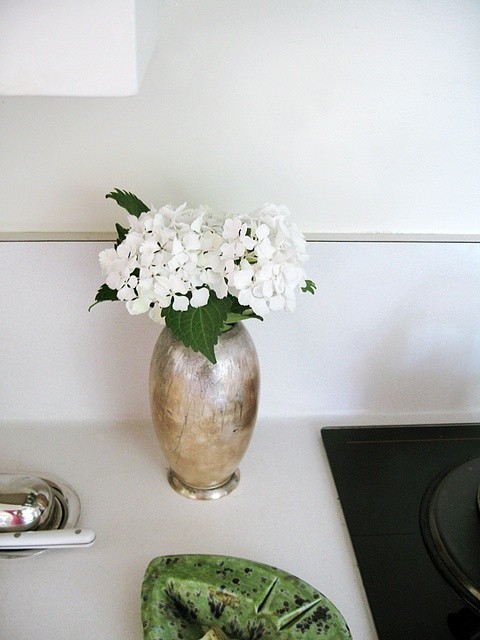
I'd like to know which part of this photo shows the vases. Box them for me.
[149,323,262,501]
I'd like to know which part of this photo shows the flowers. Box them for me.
[89,185,316,366]
